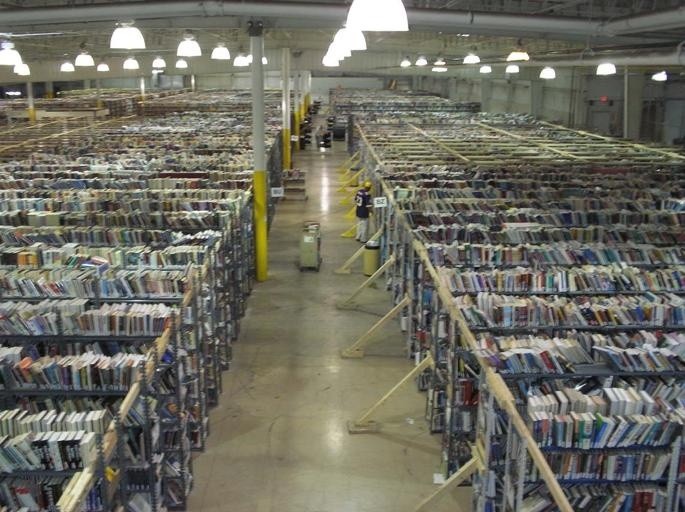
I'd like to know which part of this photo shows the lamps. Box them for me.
[0,19,250,75]
[399,38,531,69]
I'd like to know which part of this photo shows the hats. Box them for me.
[365,182,372,188]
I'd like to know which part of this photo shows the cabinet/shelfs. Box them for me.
[327,86,684,511]
[0,88,315,511]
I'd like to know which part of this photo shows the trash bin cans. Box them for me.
[351,168,359,187]
[364,246,380,277]
[299,221,320,272]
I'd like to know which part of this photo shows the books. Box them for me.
[0,88,300,512]
[327,89,683,512]
